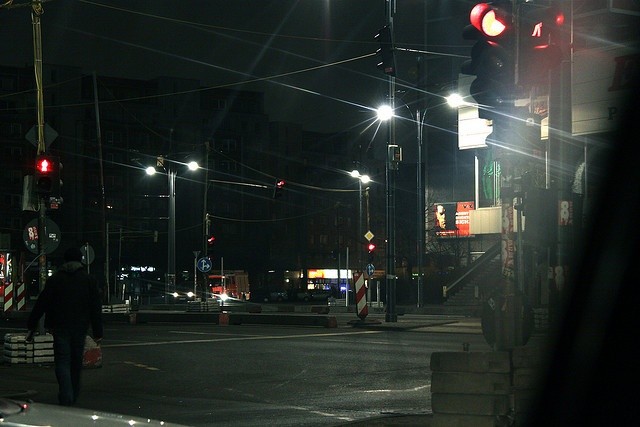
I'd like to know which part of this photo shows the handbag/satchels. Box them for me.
[81,326,104,369]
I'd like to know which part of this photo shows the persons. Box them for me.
[26,250,104,408]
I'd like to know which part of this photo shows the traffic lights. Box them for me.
[274,178,285,198]
[208,235,216,246]
[519,6,565,86]
[460,0,512,118]
[374,28,396,74]
[36,155,63,195]
[367,242,376,254]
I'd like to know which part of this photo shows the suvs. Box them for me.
[271,287,312,301]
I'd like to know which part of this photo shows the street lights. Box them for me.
[351,170,372,306]
[145,161,198,303]
[377,94,463,321]
